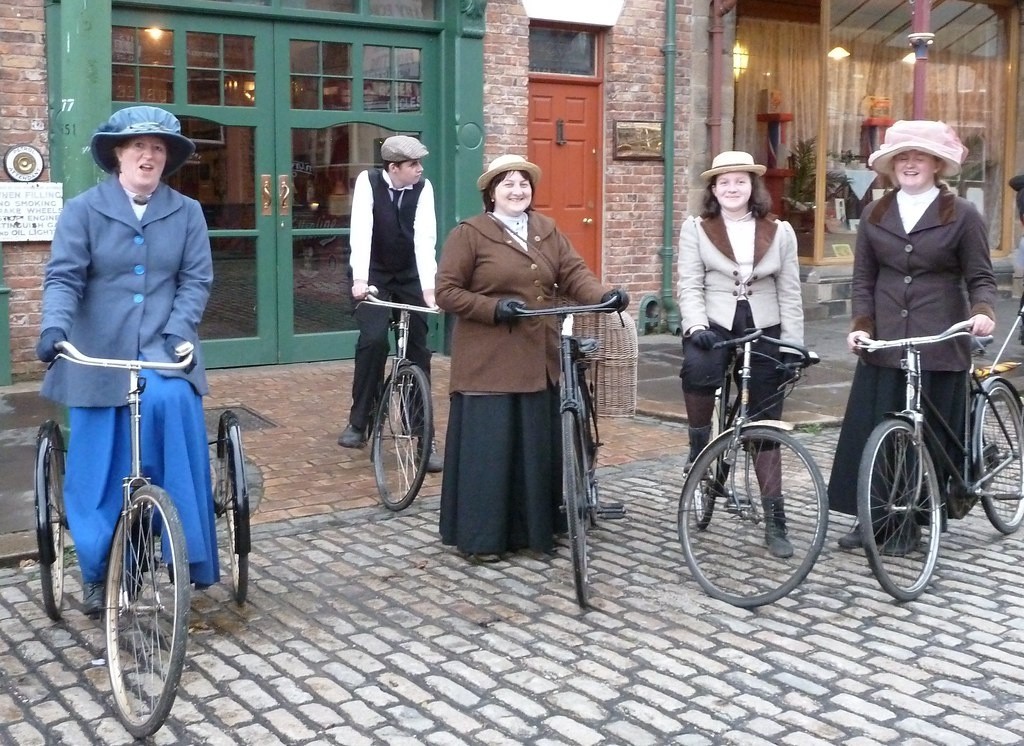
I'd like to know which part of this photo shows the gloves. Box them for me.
[600,288,631,314]
[779,353,802,382]
[497,299,527,322]
[691,328,717,349]
[166,334,198,374]
[37,326,68,363]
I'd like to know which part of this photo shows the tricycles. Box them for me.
[32,342,251,739]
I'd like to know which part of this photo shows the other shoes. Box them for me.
[417,435,444,473]
[837,525,890,548]
[886,522,921,555]
[338,423,366,448]
[83,580,105,619]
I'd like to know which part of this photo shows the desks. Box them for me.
[823,168,878,217]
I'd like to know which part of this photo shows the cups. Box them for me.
[851,160,860,170]
[860,163,866,170]
[826,161,833,169]
[848,219,860,232]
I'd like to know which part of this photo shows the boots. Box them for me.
[759,495,793,559]
[683,421,712,478]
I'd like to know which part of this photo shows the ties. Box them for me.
[389,188,403,228]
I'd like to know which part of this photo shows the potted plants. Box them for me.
[780,137,861,228]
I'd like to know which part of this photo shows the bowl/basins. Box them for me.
[834,163,845,170]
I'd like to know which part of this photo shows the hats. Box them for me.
[700,151,767,180]
[91,106,196,179]
[380,135,429,162]
[868,119,970,177]
[476,154,543,191]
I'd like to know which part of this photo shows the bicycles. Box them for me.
[677,328,829,607]
[508,294,626,609]
[354,285,444,513]
[852,319,1024,601]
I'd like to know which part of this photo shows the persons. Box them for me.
[827,120,996,557]
[676,151,805,559]
[339,136,444,472]
[36,106,220,617]
[433,154,629,562]
